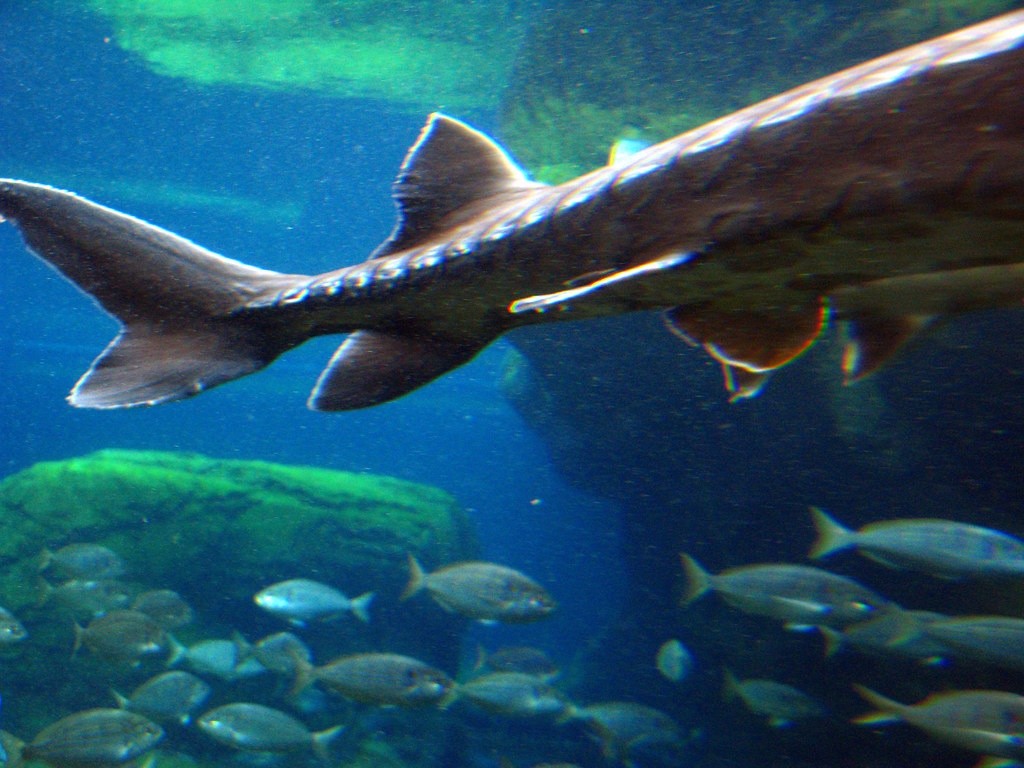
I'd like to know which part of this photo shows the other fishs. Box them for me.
[0,7,1024,768]
